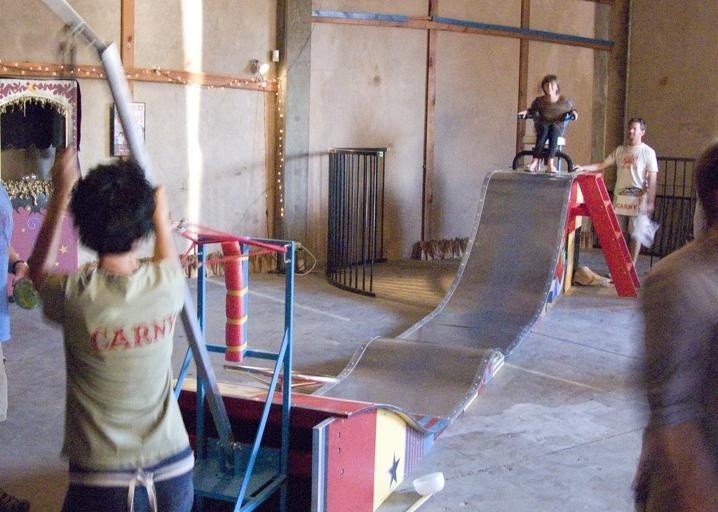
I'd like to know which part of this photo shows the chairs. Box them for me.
[513,103,576,172]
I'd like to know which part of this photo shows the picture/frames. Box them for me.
[111,102,146,157]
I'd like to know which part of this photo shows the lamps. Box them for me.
[255,59,270,74]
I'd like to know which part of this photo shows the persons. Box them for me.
[573,117,660,268]
[28,145,195,511]
[0,179,33,428]
[512,73,579,175]
[632,139,717,511]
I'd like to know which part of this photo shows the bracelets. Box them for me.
[12,260,26,272]
